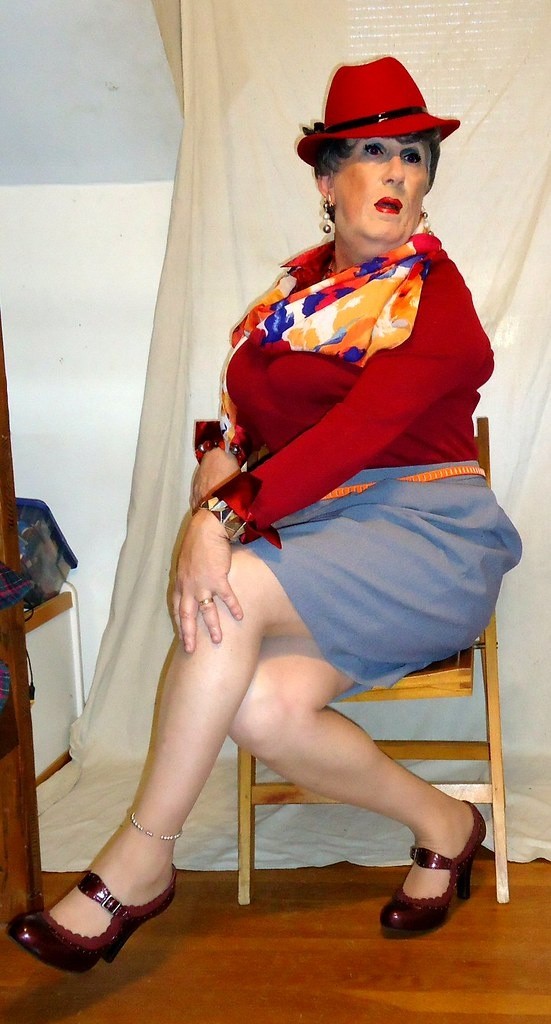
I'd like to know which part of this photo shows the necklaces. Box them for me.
[324,259,341,278]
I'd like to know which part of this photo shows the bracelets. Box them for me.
[195,440,243,463]
[200,496,246,541]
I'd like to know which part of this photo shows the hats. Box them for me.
[297,58,460,167]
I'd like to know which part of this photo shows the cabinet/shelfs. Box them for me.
[16,581,86,814]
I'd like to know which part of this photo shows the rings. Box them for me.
[199,597,214,605]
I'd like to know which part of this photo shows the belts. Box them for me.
[320,464,487,501]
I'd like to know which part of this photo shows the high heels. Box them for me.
[378,799,485,938]
[7,863,178,973]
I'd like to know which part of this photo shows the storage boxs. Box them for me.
[16,497,78,609]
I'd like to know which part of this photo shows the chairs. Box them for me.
[237,418,509,905]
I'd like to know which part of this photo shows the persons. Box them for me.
[7,58,522,972]
